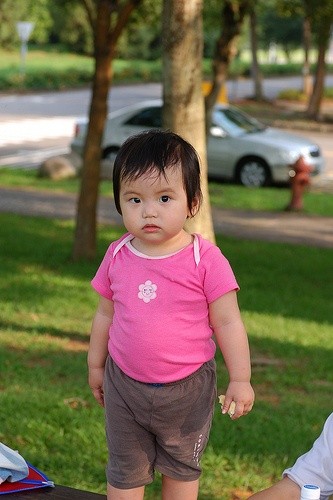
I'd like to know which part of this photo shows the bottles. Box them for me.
[300,484,320,500]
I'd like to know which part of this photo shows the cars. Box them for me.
[69,99,323,189]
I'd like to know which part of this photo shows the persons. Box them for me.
[88,131,254,500]
[247,412,333,500]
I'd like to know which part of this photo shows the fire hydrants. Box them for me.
[282,152,315,213]
